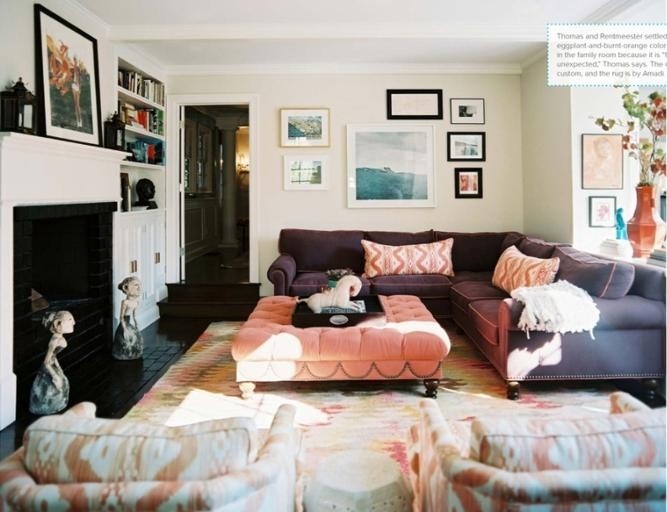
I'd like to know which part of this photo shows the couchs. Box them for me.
[405,390,665,511]
[267,228,665,400]
[1,401,297,512]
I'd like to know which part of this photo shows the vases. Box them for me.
[626,184,665,265]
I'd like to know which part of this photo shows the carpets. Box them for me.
[121,321,552,512]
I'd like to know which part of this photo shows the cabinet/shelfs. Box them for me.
[99,41,167,331]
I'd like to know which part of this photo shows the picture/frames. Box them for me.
[386,89,443,120]
[454,168,483,198]
[450,98,485,124]
[346,123,437,208]
[581,133,624,190]
[279,107,331,148]
[33,3,104,148]
[284,155,328,191]
[447,131,486,162]
[589,197,617,227]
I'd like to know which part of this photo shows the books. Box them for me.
[118,71,166,165]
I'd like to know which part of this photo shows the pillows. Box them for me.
[491,244,560,293]
[23,417,258,483]
[549,246,636,299]
[517,236,572,259]
[465,408,665,473]
[360,237,455,277]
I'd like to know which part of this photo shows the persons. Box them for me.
[71,57,83,128]
[111,276,144,360]
[135,179,158,209]
[29,309,77,415]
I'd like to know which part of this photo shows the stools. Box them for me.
[303,449,415,512]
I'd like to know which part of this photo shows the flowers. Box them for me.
[585,85,666,187]
